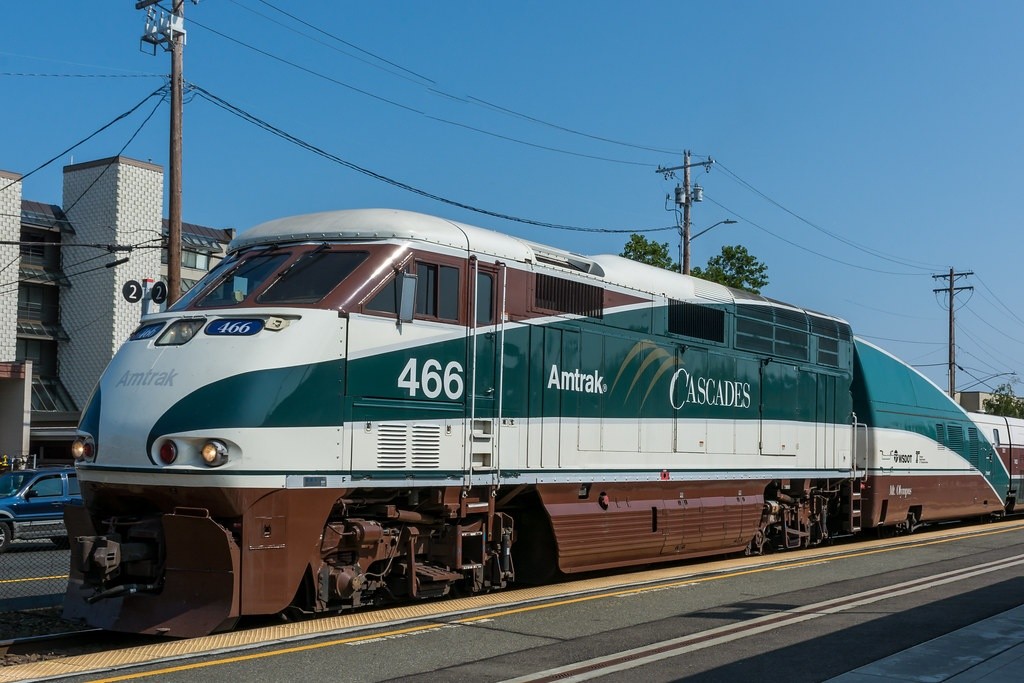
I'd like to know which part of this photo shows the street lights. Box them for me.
[684,219,739,276]
[949,372,1018,402]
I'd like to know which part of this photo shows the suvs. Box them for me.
[0,464,83,552]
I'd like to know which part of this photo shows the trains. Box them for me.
[60,208,1024,639]
